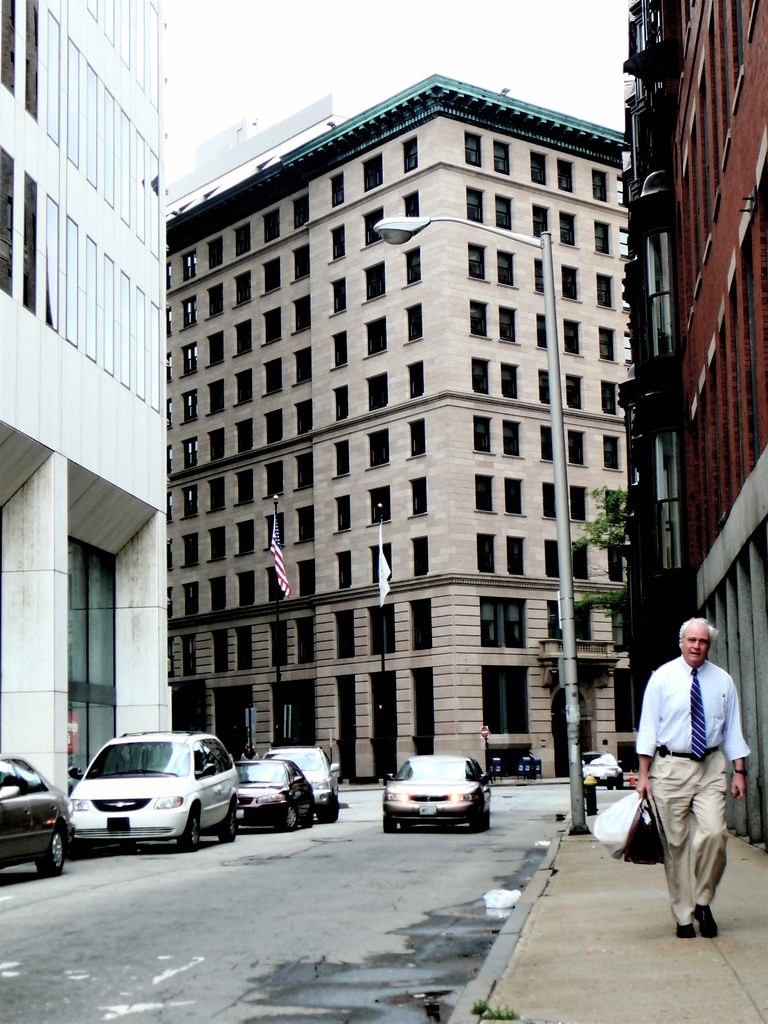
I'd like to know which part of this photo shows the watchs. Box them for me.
[734,770,748,777]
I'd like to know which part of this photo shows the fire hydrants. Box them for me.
[582,774,598,815]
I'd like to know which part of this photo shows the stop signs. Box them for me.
[479,725,489,738]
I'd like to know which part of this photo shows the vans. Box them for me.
[68,730,238,852]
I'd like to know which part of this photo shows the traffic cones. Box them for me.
[629,770,637,787]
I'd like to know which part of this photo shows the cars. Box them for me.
[383,756,492,832]
[0,755,75,876]
[234,759,314,831]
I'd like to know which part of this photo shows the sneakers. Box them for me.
[694,904,717,939]
[676,922,696,938]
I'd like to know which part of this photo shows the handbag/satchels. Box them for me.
[593,789,665,865]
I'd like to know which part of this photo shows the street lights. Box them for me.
[374,216,592,835]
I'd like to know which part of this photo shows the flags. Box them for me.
[270,511,291,599]
[379,521,392,607]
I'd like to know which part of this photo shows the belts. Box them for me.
[656,746,719,759]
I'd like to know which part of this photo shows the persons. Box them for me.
[636,617,751,939]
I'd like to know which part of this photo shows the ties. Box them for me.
[690,669,706,758]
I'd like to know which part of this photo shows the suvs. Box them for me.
[261,745,340,823]
[578,750,624,790]
